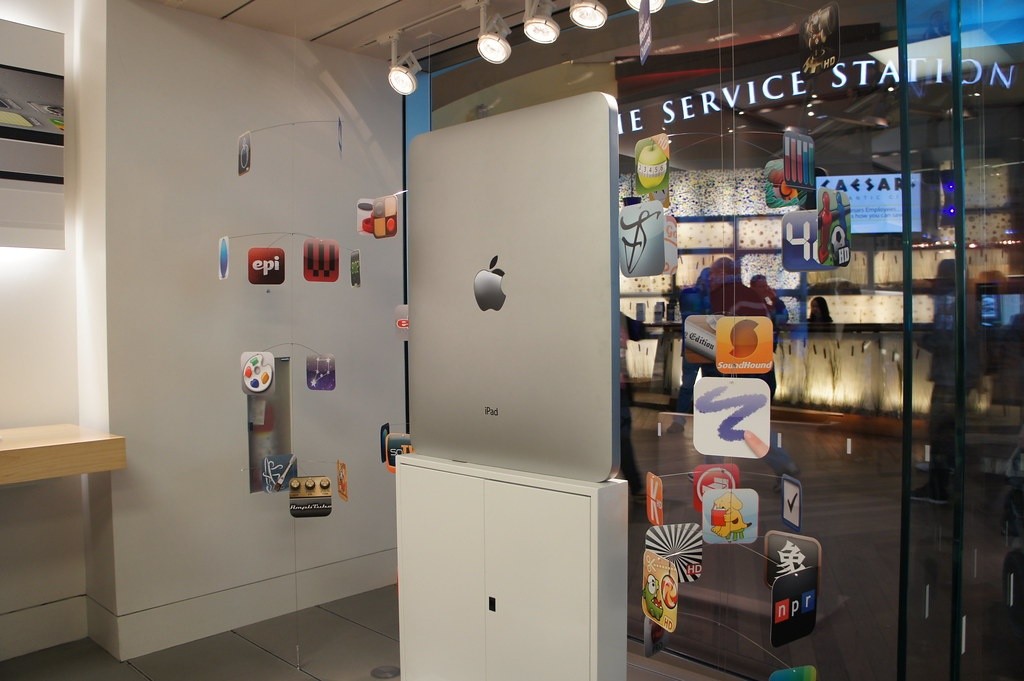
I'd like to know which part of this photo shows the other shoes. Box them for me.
[667,422,684,433]
[634,489,646,504]
[773,470,800,492]
[911,483,949,504]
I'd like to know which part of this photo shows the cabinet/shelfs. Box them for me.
[395,453,628,681]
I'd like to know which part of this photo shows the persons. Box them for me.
[911,260,1006,504]
[667,257,799,494]
[806,297,833,322]
[619,313,651,510]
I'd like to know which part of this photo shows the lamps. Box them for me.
[523,0,560,43]
[569,0,608,29]
[625,0,667,14]
[461,0,511,65]
[377,29,422,96]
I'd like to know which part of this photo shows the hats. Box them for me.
[923,260,969,282]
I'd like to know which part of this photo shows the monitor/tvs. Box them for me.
[815,169,941,251]
